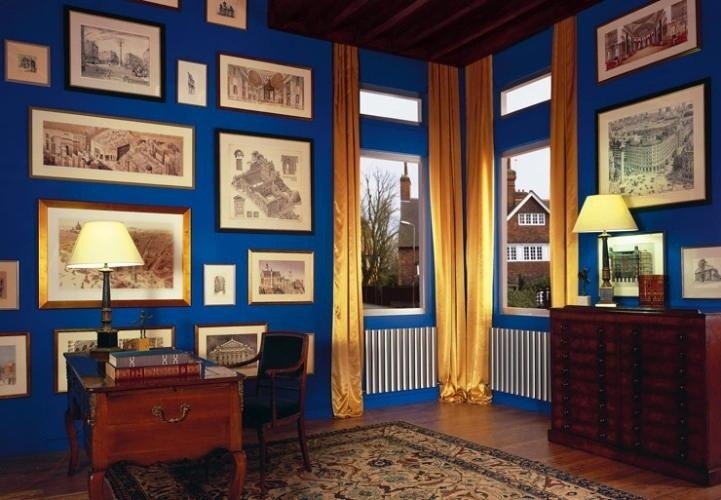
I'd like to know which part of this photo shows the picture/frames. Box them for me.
[595,0,702,85]
[597,230,668,299]
[595,76,712,213]
[1,261,30,400]
[215,127,314,233]
[194,323,268,379]
[203,264,236,307]
[36,197,192,307]
[248,249,314,305]
[306,333,316,375]
[680,244,721,299]
[54,326,175,395]
[28,107,197,191]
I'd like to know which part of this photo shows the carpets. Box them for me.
[99,420,648,500]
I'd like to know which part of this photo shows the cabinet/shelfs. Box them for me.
[547,306,721,486]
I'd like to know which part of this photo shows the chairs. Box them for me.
[205,332,312,495]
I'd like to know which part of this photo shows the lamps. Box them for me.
[572,194,638,308]
[66,219,146,363]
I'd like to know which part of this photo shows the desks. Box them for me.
[63,349,248,500]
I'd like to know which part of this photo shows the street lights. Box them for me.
[399,221,418,306]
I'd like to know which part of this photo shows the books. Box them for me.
[105,348,202,383]
